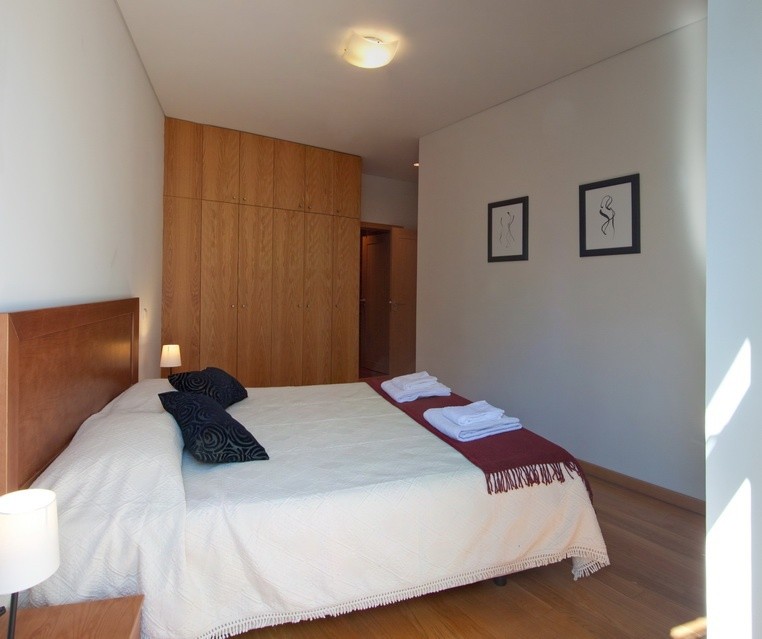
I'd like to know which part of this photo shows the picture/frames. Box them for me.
[577,174,641,258]
[486,196,528,263]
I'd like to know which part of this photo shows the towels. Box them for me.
[442,399,505,426]
[380,380,451,403]
[391,370,438,391]
[422,408,523,443]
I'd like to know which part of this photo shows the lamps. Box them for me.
[0,489,60,639]
[159,345,182,375]
[344,32,398,68]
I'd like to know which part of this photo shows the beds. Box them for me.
[0,297,611,639]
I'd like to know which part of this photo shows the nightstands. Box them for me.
[0,593,145,639]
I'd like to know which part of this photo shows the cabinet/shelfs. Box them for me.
[158,116,362,385]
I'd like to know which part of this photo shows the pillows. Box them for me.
[168,366,249,408]
[100,378,175,414]
[157,391,270,464]
[30,410,183,512]
